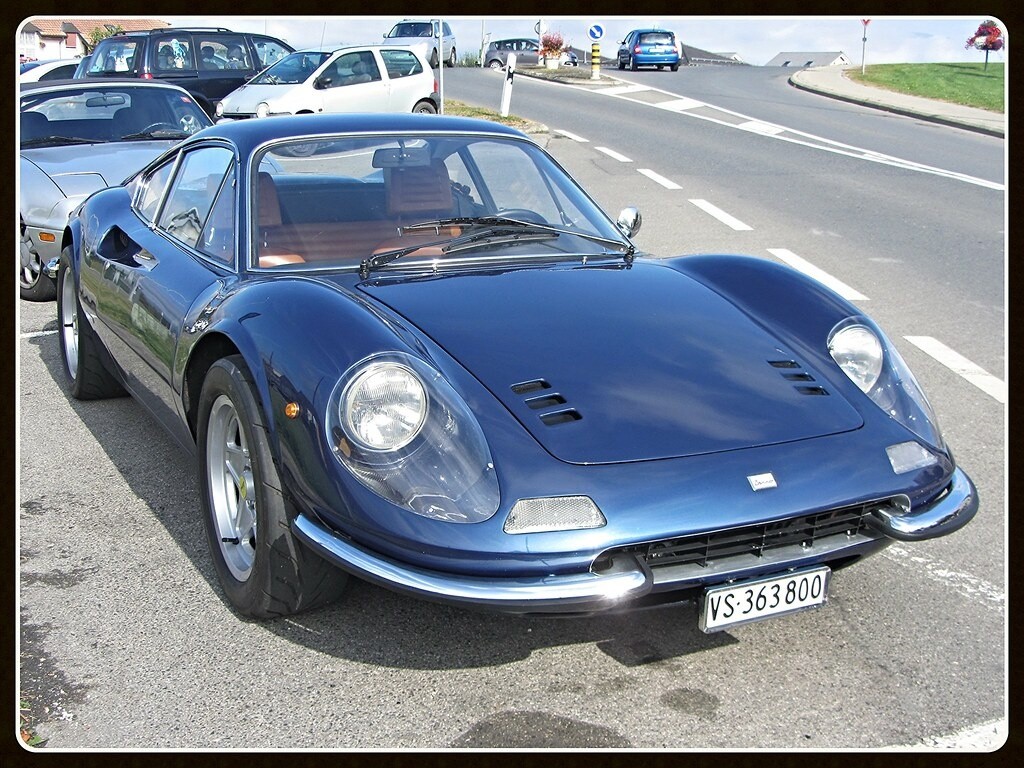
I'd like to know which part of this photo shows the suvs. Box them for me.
[376,17,458,68]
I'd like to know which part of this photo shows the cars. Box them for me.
[614,27,682,72]
[19,76,216,303]
[20,56,83,84]
[49,112,979,635]
[212,43,442,125]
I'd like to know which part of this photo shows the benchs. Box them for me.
[257,218,462,262]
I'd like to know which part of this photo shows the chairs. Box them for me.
[372,157,464,256]
[341,60,373,86]
[321,62,340,86]
[401,26,414,36]
[201,46,220,71]
[224,45,248,69]
[207,170,307,267]
[21,112,52,142]
[111,107,152,140]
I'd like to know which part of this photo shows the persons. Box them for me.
[418,26,431,36]
[166,52,176,69]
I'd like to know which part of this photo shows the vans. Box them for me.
[483,36,578,70]
[71,27,319,116]
